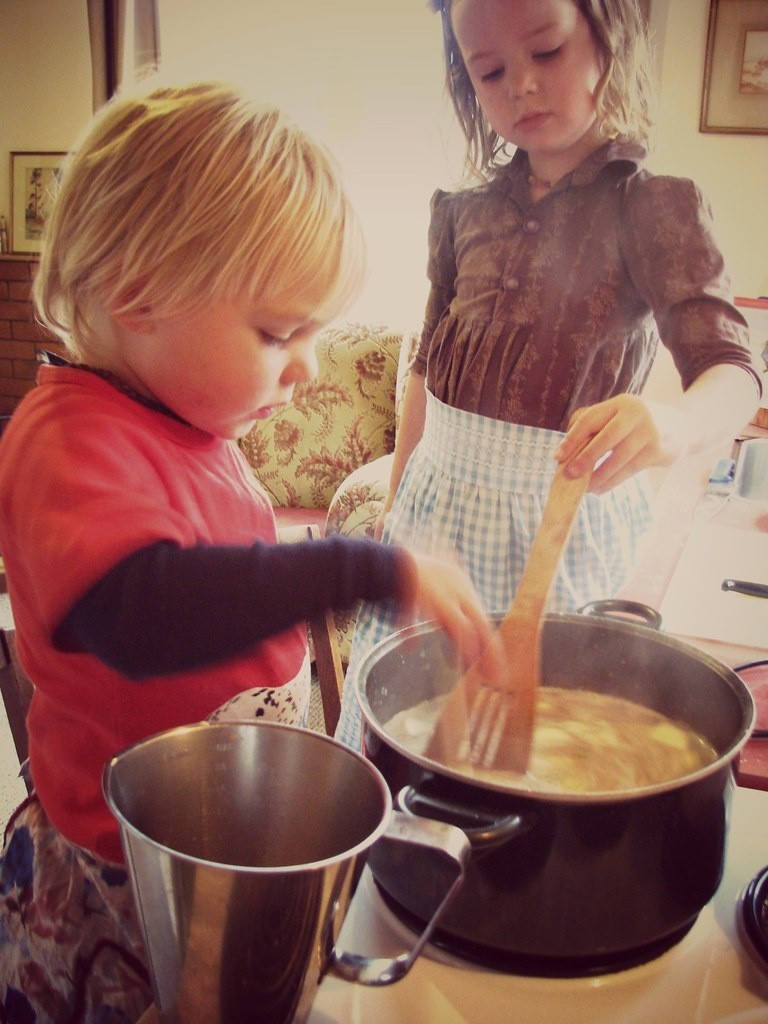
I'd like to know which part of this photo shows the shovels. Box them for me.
[423,409,595,776]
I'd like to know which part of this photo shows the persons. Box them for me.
[332,0,767,764]
[0,66,502,1024]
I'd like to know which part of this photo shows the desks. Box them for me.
[133,784,767,1024]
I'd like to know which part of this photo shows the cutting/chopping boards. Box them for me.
[656,523,768,652]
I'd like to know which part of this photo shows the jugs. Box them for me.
[100,717,475,1024]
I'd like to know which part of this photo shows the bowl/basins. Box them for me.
[740,864,768,964]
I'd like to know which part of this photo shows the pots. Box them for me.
[352,599,758,965]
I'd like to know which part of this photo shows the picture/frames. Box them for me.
[699,0,768,135]
[9,151,69,256]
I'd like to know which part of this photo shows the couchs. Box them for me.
[243,322,420,670]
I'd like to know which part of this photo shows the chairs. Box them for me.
[731,437,768,499]
